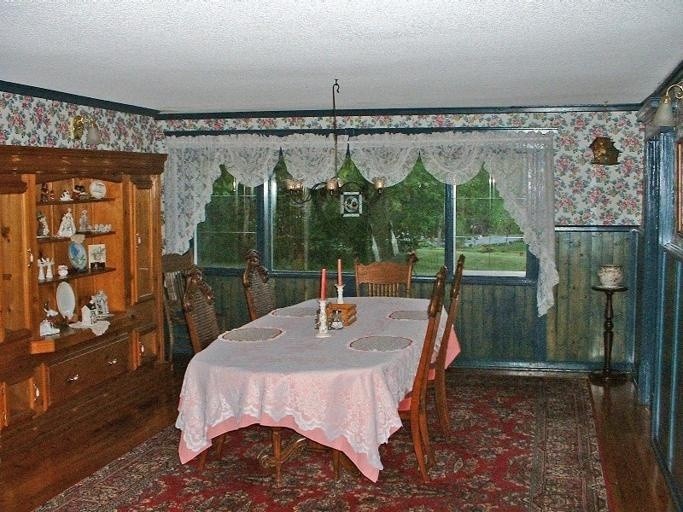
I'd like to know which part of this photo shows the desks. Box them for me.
[590,284,627,388]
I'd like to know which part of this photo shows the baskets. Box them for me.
[315,302,358,327]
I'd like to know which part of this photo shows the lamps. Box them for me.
[651,80,683,127]
[277,76,389,205]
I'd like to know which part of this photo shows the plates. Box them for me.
[67,241,87,270]
[54,281,75,320]
[88,181,106,200]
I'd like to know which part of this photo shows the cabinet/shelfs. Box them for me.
[1,144,179,512]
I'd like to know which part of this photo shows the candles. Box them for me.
[321,268,327,301]
[337,259,343,286]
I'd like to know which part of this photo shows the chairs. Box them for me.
[244,248,282,324]
[355,254,418,295]
[405,253,468,443]
[166,268,317,487]
[334,265,449,487]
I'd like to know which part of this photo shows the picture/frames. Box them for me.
[341,191,363,218]
[87,244,107,269]
